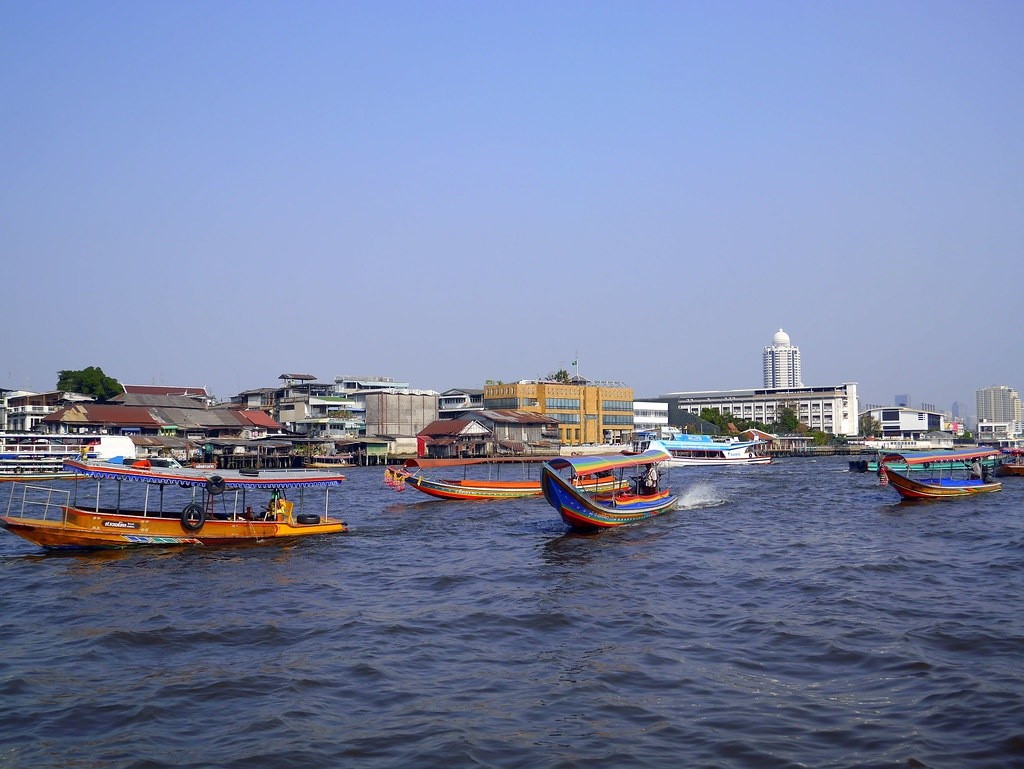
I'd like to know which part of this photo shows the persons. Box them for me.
[961,458,981,480]
[571,452,582,480]
[641,464,657,494]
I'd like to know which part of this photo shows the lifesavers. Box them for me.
[206,476,226,495]
[749,454,752,458]
[296,513,321,524]
[238,468,260,476]
[924,462,930,468]
[181,504,206,532]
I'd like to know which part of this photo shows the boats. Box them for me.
[849,449,1011,473]
[0,459,350,552]
[620,435,773,468]
[538,447,679,530]
[304,454,358,468]
[875,446,1002,501]
[382,453,630,501]
[991,438,1024,478]
